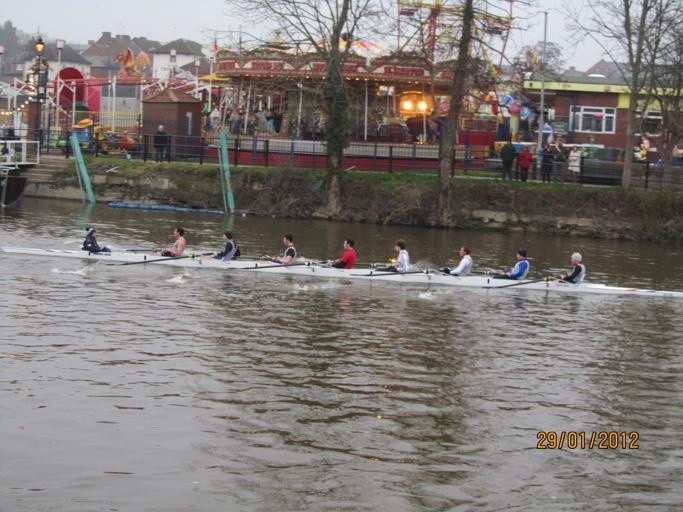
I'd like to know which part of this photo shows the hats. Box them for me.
[517,250,527,256]
[88,228,95,234]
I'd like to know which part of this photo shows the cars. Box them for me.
[561,142,683,179]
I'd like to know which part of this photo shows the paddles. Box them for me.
[350,267,446,277]
[227,262,329,269]
[105,250,218,267]
[481,273,570,289]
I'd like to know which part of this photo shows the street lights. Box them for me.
[33,35,45,157]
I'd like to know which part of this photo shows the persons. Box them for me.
[560,252,585,284]
[640,133,650,150]
[444,245,473,276]
[493,248,530,280]
[153,125,167,161]
[500,138,582,184]
[326,238,357,269]
[213,231,240,261]
[82,227,112,256]
[153,226,186,257]
[385,239,410,273]
[271,233,297,265]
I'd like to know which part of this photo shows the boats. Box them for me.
[0,245,683,301]
